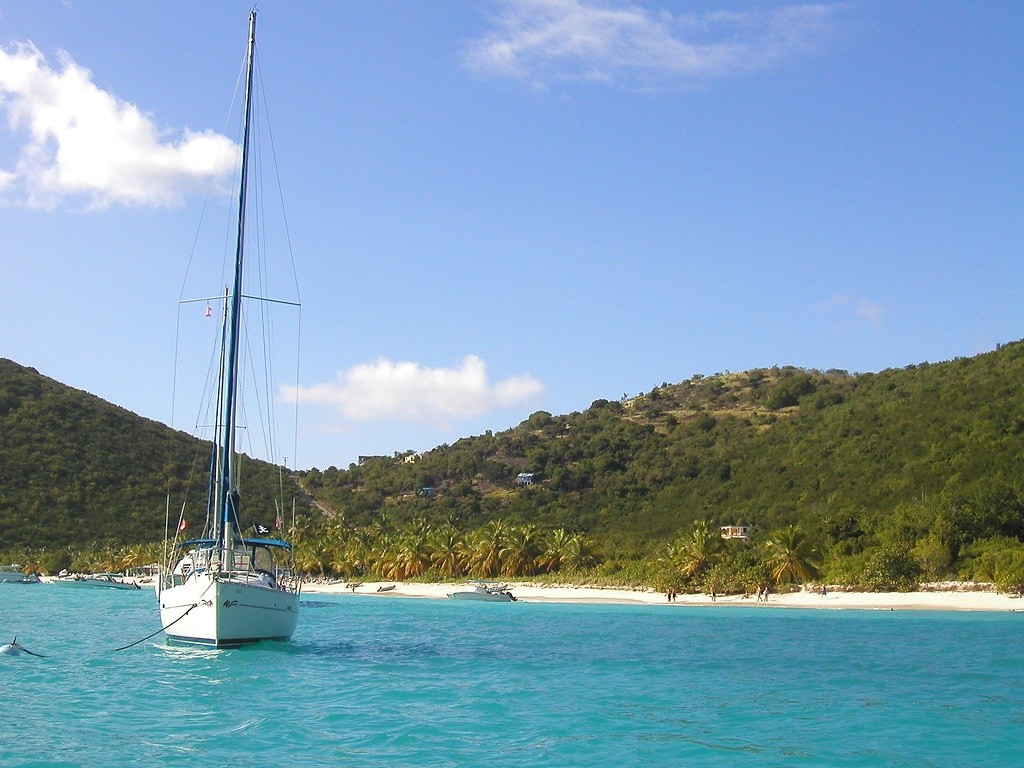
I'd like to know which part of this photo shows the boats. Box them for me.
[447,585,511,602]
[49,576,141,590]
[0,566,42,583]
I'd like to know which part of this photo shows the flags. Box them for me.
[253,522,272,535]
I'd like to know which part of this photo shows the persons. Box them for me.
[820,584,827,598]
[756,585,763,602]
[665,587,676,603]
[710,585,716,601]
[763,586,769,602]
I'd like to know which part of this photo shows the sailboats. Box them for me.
[159,3,304,651]
